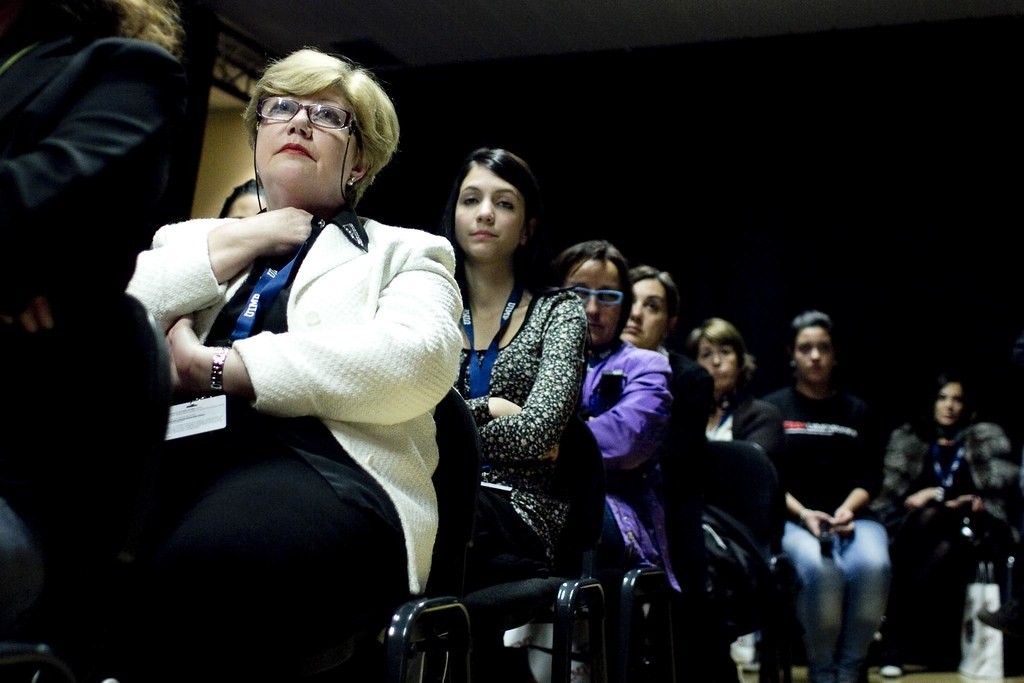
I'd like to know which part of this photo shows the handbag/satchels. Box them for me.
[959,562,1005,681]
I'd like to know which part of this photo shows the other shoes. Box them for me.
[879,655,902,676]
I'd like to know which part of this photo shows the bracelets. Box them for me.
[209,347,230,395]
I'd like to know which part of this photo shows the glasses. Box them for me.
[554,284,626,305]
[255,97,364,152]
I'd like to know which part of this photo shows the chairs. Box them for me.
[0,442,785,683]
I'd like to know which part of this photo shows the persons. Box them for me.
[0,0,194,683]
[434,148,588,683]
[554,240,1024,683]
[219,179,260,219]
[58,49,469,683]
